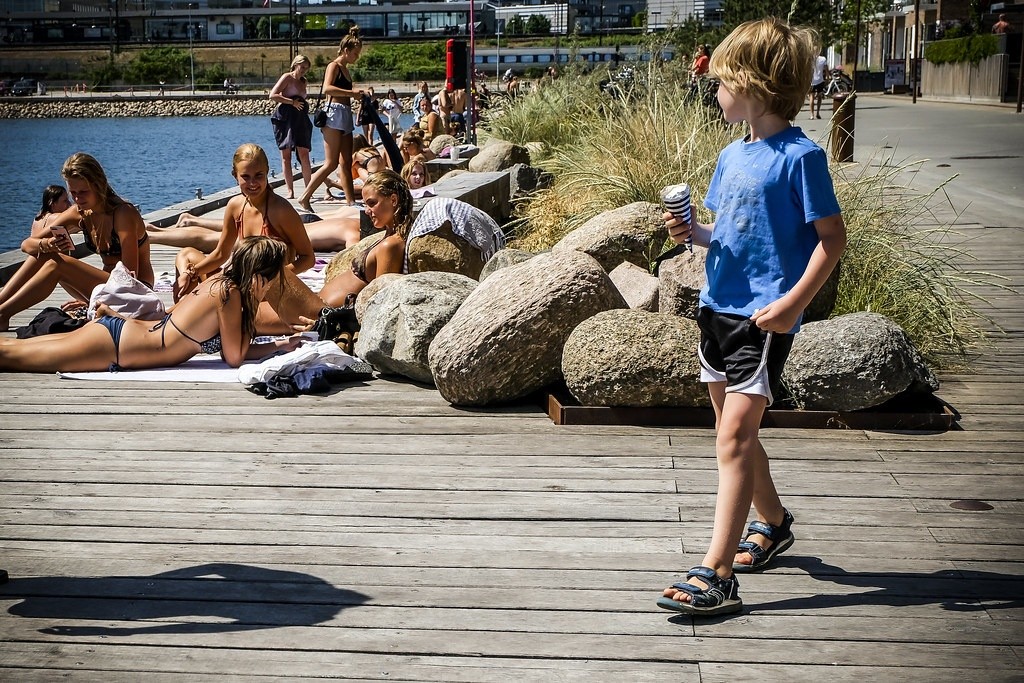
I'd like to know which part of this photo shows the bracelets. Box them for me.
[184,262,199,282]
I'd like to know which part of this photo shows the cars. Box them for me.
[0,79,38,97]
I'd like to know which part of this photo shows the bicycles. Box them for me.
[817,70,848,96]
[219,86,238,95]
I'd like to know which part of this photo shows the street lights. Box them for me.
[480,2,502,93]
[187,3,197,94]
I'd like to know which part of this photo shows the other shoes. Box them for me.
[816,115,822,119]
[808,117,814,120]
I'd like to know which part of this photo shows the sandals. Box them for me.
[732,507,795,574]
[656,565,743,616]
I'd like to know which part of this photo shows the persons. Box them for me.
[0,234,311,372]
[30,185,71,237]
[991,14,1009,34]
[507,76,518,92]
[808,50,829,120]
[689,44,710,93]
[471,82,489,110]
[401,160,430,188]
[0,80,13,96]
[171,143,315,304]
[175,208,360,232]
[548,65,557,77]
[158,78,165,96]
[297,35,364,211]
[269,55,313,198]
[475,70,488,85]
[224,78,236,94]
[144,218,360,252]
[253,170,414,334]
[0,152,154,333]
[323,80,469,198]
[505,68,512,83]
[657,17,846,615]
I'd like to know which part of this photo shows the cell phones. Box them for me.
[50,226,75,251]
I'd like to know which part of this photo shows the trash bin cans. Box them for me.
[37,82,46,95]
[832,93,856,164]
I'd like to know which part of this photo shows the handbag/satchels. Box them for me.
[313,110,327,128]
[310,293,361,341]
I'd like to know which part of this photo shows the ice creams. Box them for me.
[659,183,694,254]
